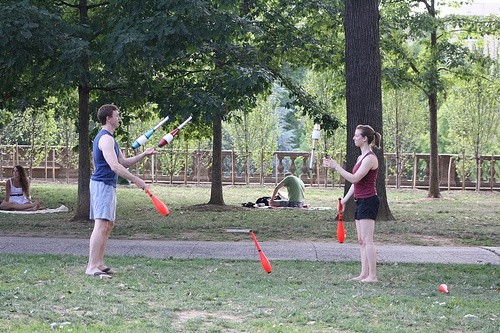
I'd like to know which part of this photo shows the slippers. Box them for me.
[89,272,112,279]
[102,267,112,274]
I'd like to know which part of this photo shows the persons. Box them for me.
[269,172,305,208]
[0,165,41,211]
[324,124,381,282]
[85,104,159,278]
